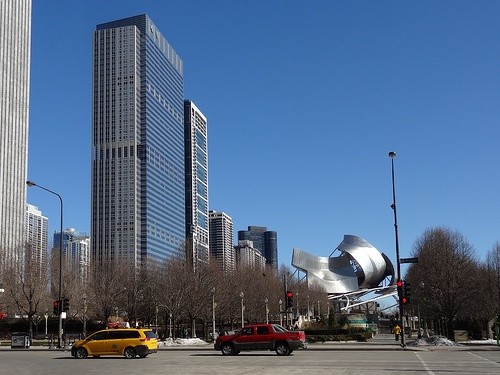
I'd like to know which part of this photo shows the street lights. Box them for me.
[295,292,299,318]
[239,291,244,328]
[82,292,87,338]
[25,180,64,348]
[318,300,321,318]
[306,294,310,321]
[279,299,283,327]
[211,286,215,342]
[326,301,330,317]
[389,150,406,347]
[397,279,405,297]
[264,298,269,324]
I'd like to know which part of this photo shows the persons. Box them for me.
[389,322,401,342]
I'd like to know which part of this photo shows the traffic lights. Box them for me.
[287,291,293,307]
[401,297,407,305]
[404,281,412,305]
[53,301,60,315]
[63,298,69,312]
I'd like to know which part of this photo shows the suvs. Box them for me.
[214,324,308,357]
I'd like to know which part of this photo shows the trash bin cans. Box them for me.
[11,332,31,349]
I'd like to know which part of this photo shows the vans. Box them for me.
[71,322,159,359]
